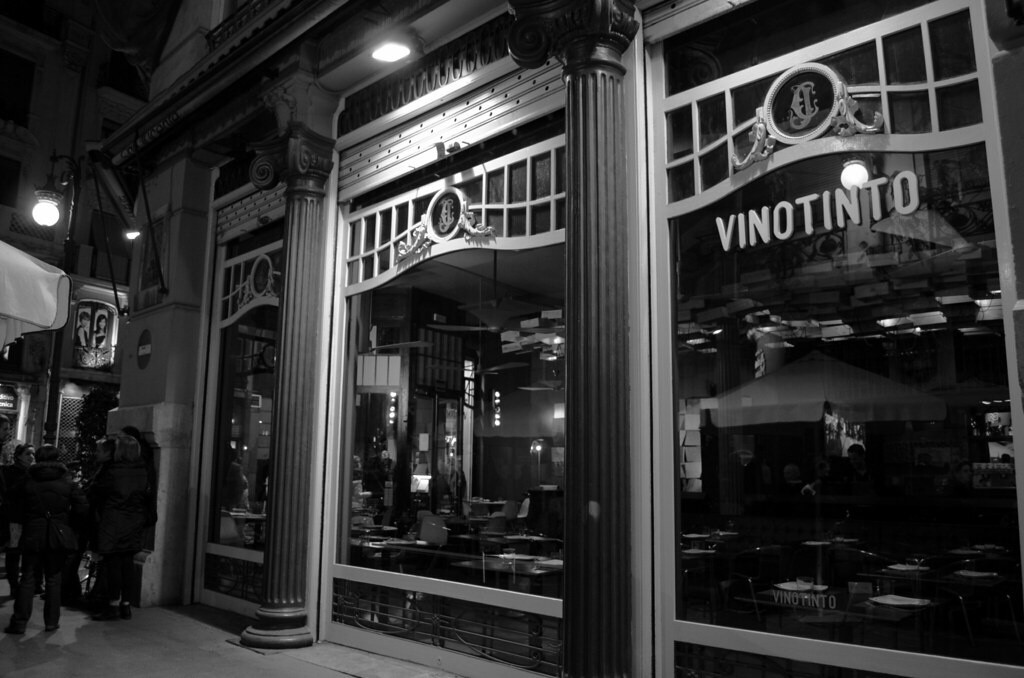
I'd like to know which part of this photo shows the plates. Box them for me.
[774,581,829,592]
[801,537,859,546]
[948,545,1001,555]
[887,563,929,571]
[868,594,931,609]
[953,570,998,578]
[680,531,738,555]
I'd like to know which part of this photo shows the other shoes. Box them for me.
[91,605,122,620]
[31,581,47,597]
[44,624,60,631]
[120,601,133,620]
[4,624,26,634]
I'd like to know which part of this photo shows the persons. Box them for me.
[76,311,107,348]
[0,426,157,635]
[781,445,979,582]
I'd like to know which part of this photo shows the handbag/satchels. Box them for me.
[44,511,79,554]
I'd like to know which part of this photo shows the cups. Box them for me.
[503,548,516,565]
[797,576,813,599]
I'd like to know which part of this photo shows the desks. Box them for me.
[215,490,1024,678]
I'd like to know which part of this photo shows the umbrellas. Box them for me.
[707,351,946,617]
